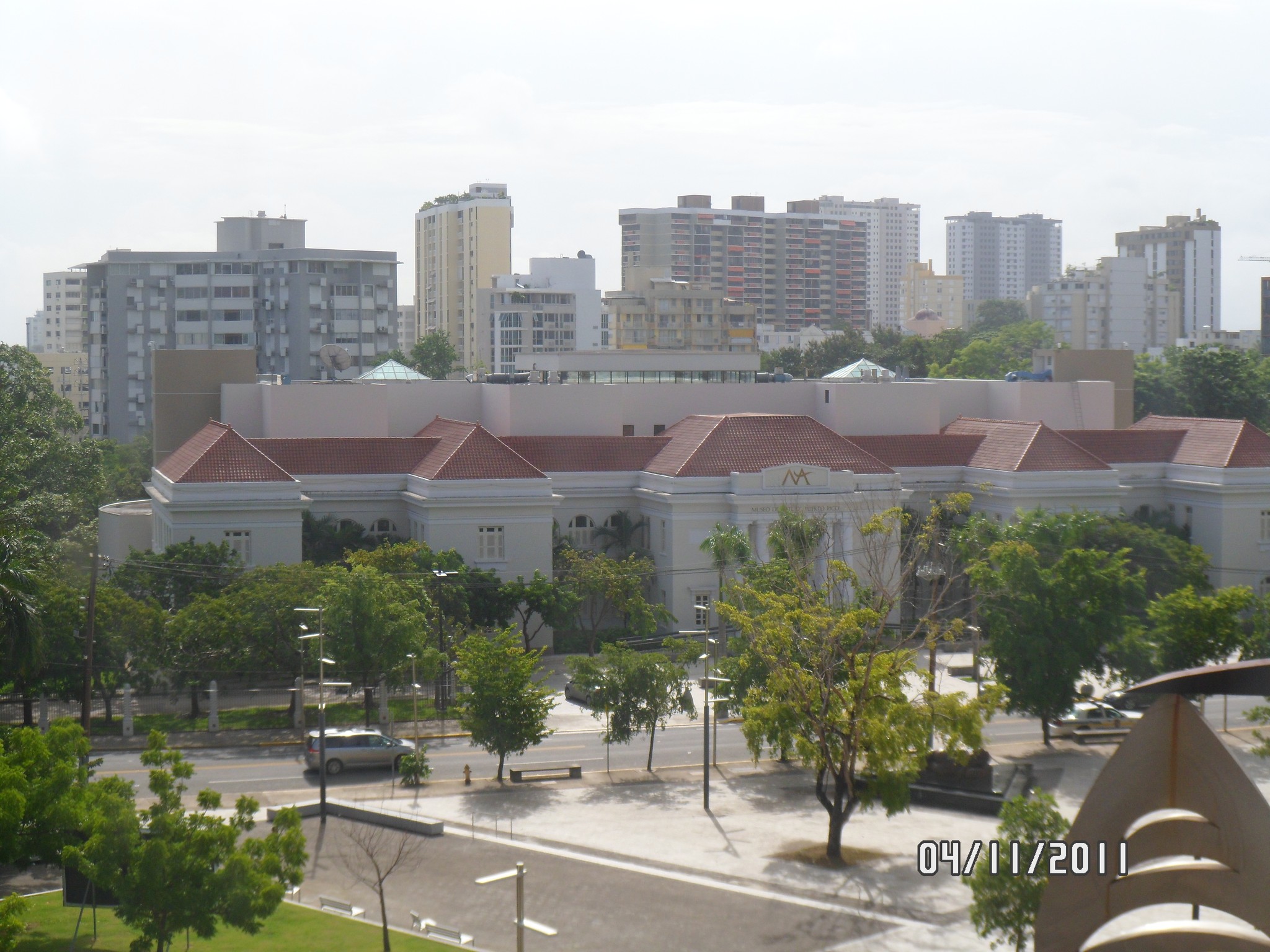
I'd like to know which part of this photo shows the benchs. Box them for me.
[510,764,581,783]
[1072,728,1131,744]
[424,924,474,947]
[698,678,715,687]
[319,896,365,919]
[948,665,973,676]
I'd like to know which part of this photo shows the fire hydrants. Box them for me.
[463,763,472,783]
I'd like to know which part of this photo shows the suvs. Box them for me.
[304,725,419,775]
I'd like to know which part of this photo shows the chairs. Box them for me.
[284,881,300,903]
[409,912,436,933]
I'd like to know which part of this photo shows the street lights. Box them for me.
[676,603,709,809]
[404,652,422,787]
[297,603,328,825]
[428,567,458,716]
[298,621,311,742]
[969,624,982,702]
[707,637,732,767]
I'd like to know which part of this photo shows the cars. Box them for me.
[1042,699,1144,737]
[1101,681,1150,710]
[565,671,622,709]
